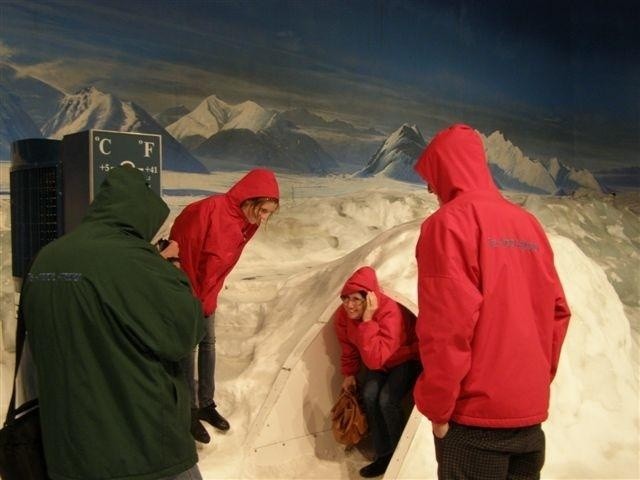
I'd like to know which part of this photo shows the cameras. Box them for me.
[155,238,171,252]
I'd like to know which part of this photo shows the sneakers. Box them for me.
[191,408,211,443]
[200,401,229,429]
[359,454,389,477]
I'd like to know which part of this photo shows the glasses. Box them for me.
[340,296,366,307]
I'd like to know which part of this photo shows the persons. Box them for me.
[331,267,422,478]
[412,122,572,480]
[168,168,280,444]
[22,165,206,480]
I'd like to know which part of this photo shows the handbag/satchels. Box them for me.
[1,396,45,480]
[330,388,367,446]
